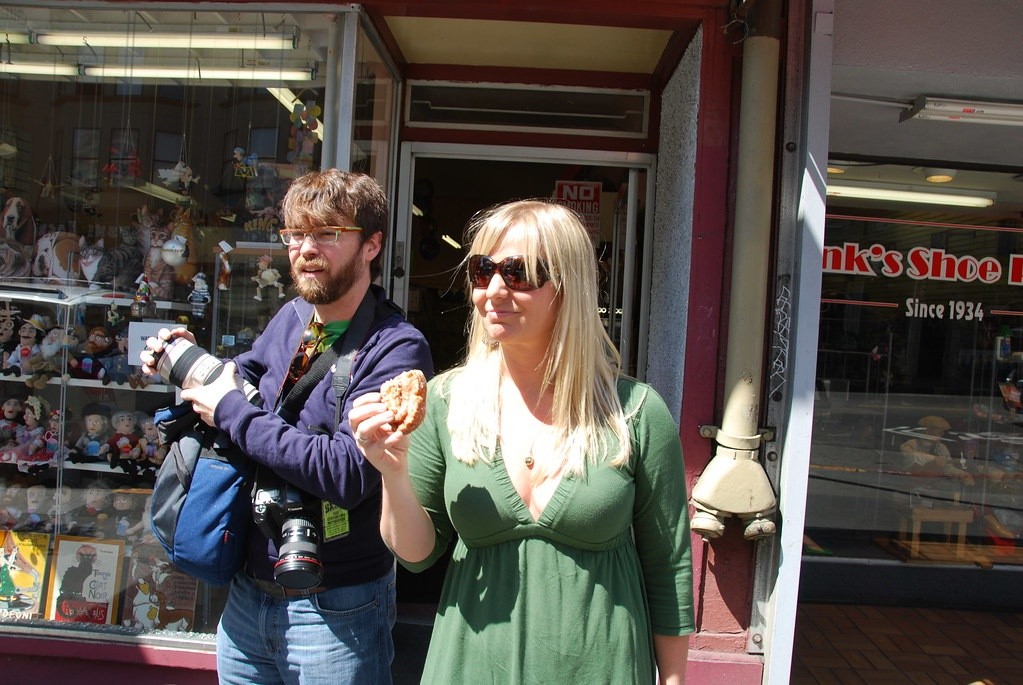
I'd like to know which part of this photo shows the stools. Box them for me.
[896,504,975,559]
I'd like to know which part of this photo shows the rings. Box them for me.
[356,433,370,443]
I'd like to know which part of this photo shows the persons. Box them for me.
[140,170,435,685]
[347,204,696,685]
[900,416,1014,539]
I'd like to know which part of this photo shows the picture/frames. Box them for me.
[44,534,126,625]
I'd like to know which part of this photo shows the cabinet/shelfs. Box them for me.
[0,280,194,559]
[971,331,1023,569]
[201,245,290,634]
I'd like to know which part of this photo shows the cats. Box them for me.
[55,551,97,619]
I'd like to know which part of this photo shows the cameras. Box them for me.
[253,488,324,591]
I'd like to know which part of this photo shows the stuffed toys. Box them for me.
[1,198,212,547]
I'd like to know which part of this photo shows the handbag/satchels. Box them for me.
[149,421,261,587]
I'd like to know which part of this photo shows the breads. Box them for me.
[380,369,427,435]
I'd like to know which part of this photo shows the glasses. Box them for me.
[287,322,334,383]
[467,255,551,291]
[280,226,361,245]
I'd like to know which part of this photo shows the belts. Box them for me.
[255,587,326,598]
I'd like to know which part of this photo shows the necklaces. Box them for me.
[524,439,537,466]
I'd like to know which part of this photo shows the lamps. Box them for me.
[27,19,302,50]
[898,94,1023,127]
[912,166,958,183]
[827,159,850,174]
[0,18,36,45]
[77,54,319,81]
[0,51,83,76]
[826,164,997,208]
[107,12,197,205]
[264,87,323,140]
